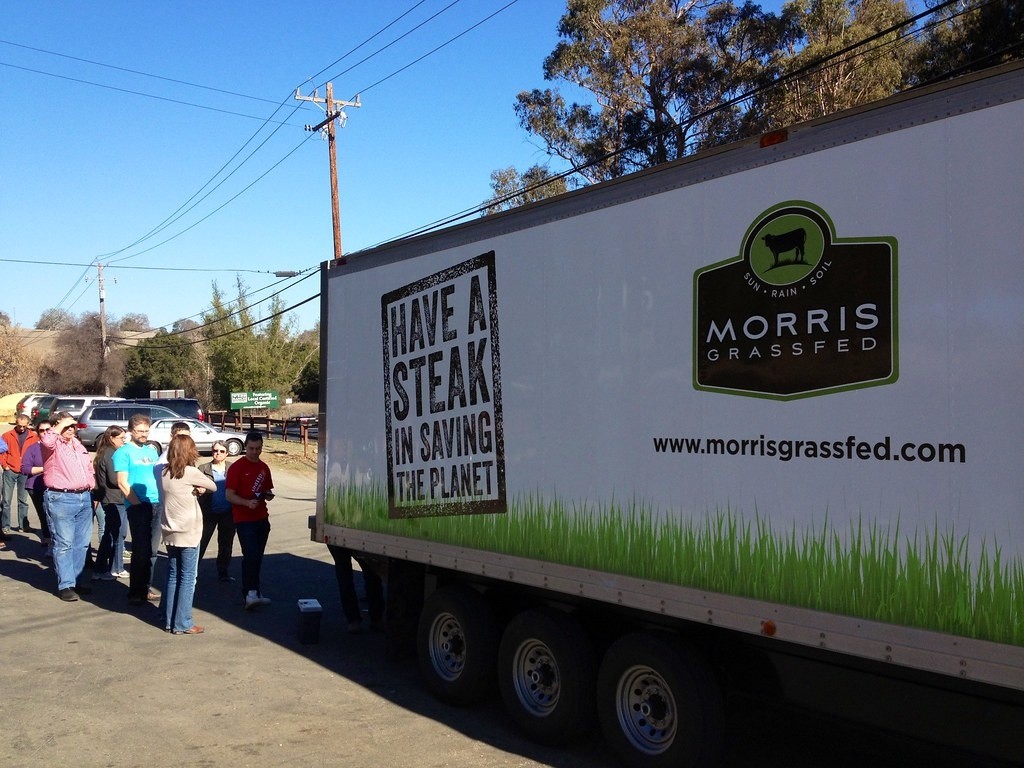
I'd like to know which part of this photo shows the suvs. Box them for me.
[49,397,127,423]
[14,394,48,419]
[113,399,204,423]
[32,396,55,426]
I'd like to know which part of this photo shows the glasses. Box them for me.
[38,428,50,432]
[213,450,225,453]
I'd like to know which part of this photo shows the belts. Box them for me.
[48,487,87,493]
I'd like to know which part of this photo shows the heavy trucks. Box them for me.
[309,58,1024,768]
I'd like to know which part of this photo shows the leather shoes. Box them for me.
[175,625,204,635]
[75,587,92,594]
[53,587,79,600]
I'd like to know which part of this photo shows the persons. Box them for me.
[160,435,218,635]
[0,411,235,601]
[225,431,276,609]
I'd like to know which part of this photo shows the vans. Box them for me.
[77,404,184,453]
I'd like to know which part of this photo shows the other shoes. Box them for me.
[19,525,37,533]
[91,571,117,580]
[245,590,272,609]
[0,540,6,549]
[128,598,154,607]
[111,570,130,578]
[347,621,360,633]
[368,619,384,630]
[219,576,236,583]
[2,528,10,534]
[147,590,161,601]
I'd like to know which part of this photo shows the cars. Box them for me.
[145,416,248,456]
